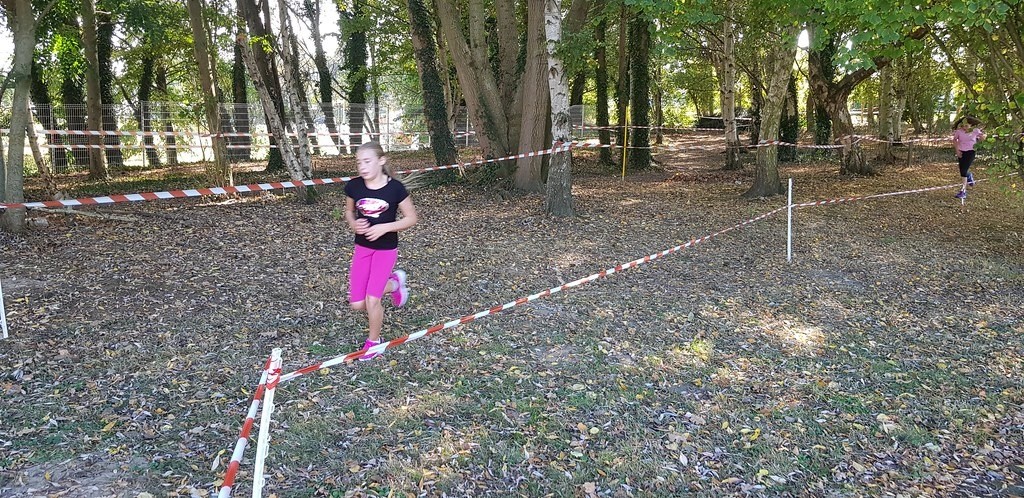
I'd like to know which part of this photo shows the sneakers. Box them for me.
[967,173,975,186]
[954,190,966,198]
[358,336,384,361]
[388,270,408,308]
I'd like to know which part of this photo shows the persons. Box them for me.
[953,117,987,198]
[344,142,418,361]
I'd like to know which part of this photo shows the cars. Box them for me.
[697,108,755,133]
